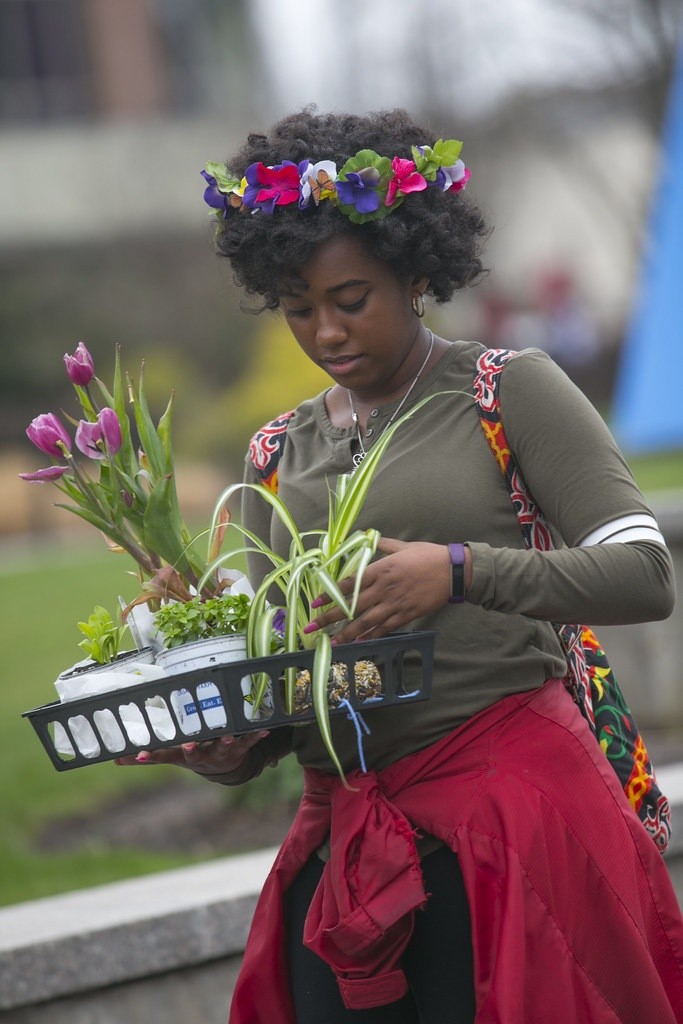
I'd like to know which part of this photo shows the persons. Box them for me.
[113,108,683,1024]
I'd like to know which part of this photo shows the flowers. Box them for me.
[202,137,471,237]
[273,609,286,632]
[17,338,235,622]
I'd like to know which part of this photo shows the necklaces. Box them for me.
[347,329,434,481]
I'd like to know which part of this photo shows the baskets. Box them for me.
[23,628,438,776]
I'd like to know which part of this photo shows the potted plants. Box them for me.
[52,389,478,792]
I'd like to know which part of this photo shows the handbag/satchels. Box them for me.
[561,620,673,855]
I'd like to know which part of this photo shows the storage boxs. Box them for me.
[22,627,438,772]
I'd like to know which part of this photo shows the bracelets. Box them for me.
[448,543,467,604]
